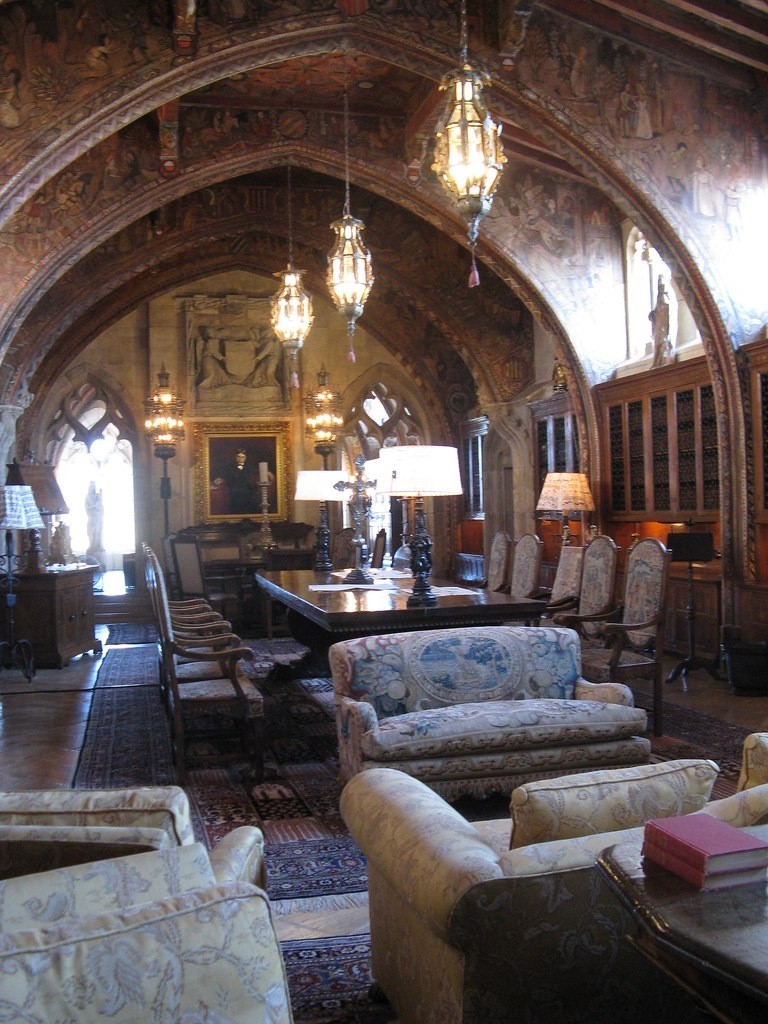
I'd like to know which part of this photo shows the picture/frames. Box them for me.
[192,416,293,528]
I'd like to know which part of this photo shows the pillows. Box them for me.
[510,759,720,848]
[735,733,767,793]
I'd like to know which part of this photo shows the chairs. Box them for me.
[141,542,266,783]
[369,528,387,568]
[484,528,672,737]
[332,525,357,569]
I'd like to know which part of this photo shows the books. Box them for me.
[642,813,768,892]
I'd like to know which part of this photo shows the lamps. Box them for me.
[12,465,70,572]
[268,162,315,390]
[0,484,47,684]
[376,446,464,607]
[303,359,343,525]
[535,472,596,544]
[326,85,374,363]
[431,0,509,286]
[141,363,186,535]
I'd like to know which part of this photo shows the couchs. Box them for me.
[339,731,768,1024]
[329,624,649,802]
[0,783,293,1024]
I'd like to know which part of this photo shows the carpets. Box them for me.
[107,621,161,643]
[72,685,749,901]
[278,932,396,1023]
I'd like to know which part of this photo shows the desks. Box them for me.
[255,569,547,674]
[596,824,767,1024]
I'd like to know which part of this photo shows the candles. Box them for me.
[259,461,267,483]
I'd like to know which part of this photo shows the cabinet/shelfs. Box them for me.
[10,566,104,669]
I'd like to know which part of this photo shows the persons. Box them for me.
[84,481,103,552]
